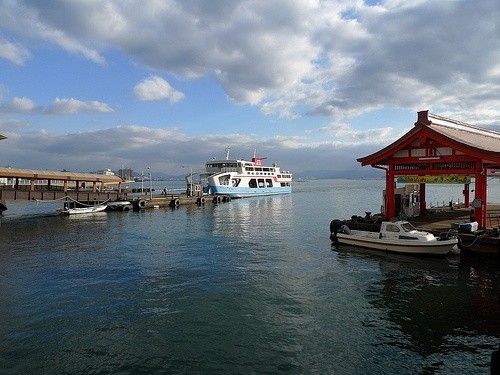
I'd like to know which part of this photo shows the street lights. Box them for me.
[182,166,192,196]
[143,168,152,200]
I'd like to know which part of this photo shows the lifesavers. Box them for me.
[223,196,231,203]
[216,196,222,202]
[122,206,130,212]
[173,198,181,206]
[138,199,146,208]
[200,197,206,204]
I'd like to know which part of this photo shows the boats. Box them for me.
[200,145,292,197]
[61,205,107,214]
[336,221,458,255]
